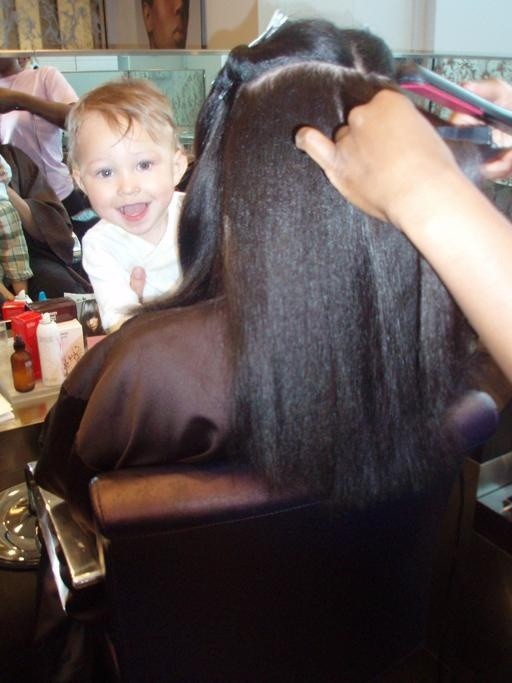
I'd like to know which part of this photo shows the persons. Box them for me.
[66,76,189,331]
[29,20,476,682]
[0,57,95,303]
[296,90,512,383]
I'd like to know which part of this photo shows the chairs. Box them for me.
[23,393,499,682]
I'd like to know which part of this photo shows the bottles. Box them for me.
[10,318,65,393]
